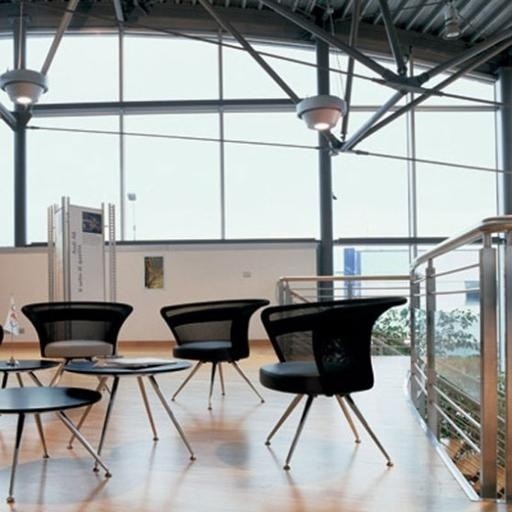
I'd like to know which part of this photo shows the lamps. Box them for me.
[440,1,462,41]
[293,2,347,133]
[2,69,50,104]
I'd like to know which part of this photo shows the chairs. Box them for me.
[257,296,406,470]
[158,298,270,413]
[22,300,134,393]
